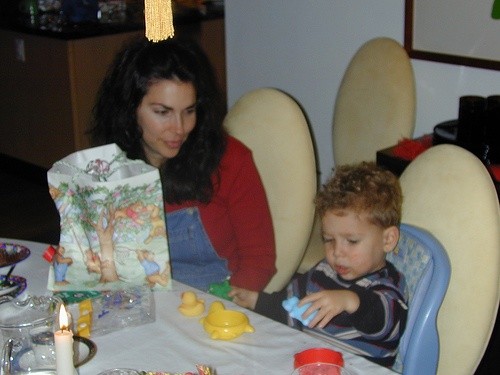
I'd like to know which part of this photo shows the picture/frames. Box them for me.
[405,0,500,71]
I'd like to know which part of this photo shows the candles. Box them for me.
[54,305,77,375]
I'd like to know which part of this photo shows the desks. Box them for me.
[0,239,402,375]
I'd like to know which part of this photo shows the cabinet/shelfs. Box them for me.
[0,14,224,168]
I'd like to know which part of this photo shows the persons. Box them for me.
[86,33,277,291]
[225,163,409,368]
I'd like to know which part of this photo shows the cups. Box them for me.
[0,294,80,375]
[293,363,353,375]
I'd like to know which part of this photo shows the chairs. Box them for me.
[332,37,417,167]
[224,88,317,292]
[398,144,500,375]
[386,224,452,375]
